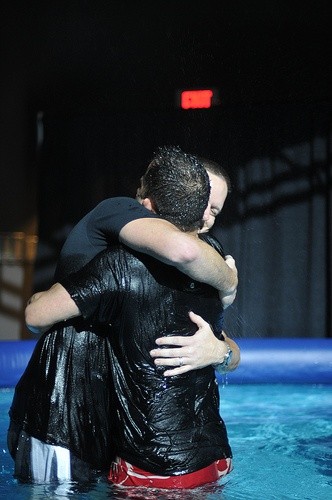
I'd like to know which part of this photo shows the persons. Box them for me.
[24,146,232,488]
[7,157,240,485]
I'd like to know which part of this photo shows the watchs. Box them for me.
[212,342,233,370]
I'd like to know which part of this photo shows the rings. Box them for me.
[179,357,183,367]
